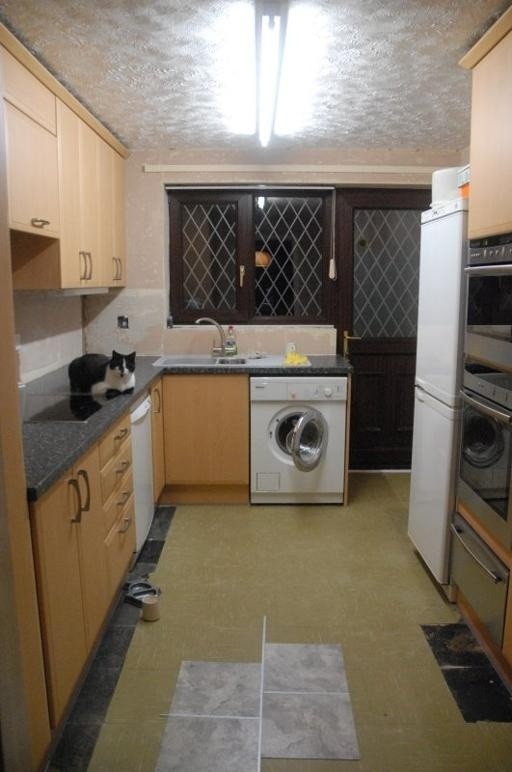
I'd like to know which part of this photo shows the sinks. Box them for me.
[218,356,247,365]
[157,355,217,366]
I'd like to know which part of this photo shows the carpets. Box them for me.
[420,622,512,723]
[151,641,359,771]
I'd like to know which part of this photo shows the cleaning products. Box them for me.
[226,325,239,355]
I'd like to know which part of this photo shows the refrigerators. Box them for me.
[405,198,468,584]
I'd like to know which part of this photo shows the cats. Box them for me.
[68,350,136,395]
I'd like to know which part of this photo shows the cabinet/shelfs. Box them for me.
[29,441,108,731]
[96,134,127,289]
[5,100,59,239]
[99,411,136,596]
[12,98,101,289]
[149,376,166,501]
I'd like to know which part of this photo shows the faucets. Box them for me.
[193,316,227,356]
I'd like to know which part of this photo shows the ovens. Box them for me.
[455,241,512,561]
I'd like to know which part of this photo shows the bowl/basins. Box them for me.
[126,581,161,608]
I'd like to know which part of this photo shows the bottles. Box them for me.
[142,596,160,622]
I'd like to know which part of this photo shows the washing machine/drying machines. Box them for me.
[456,370,512,501]
[248,373,349,506]
